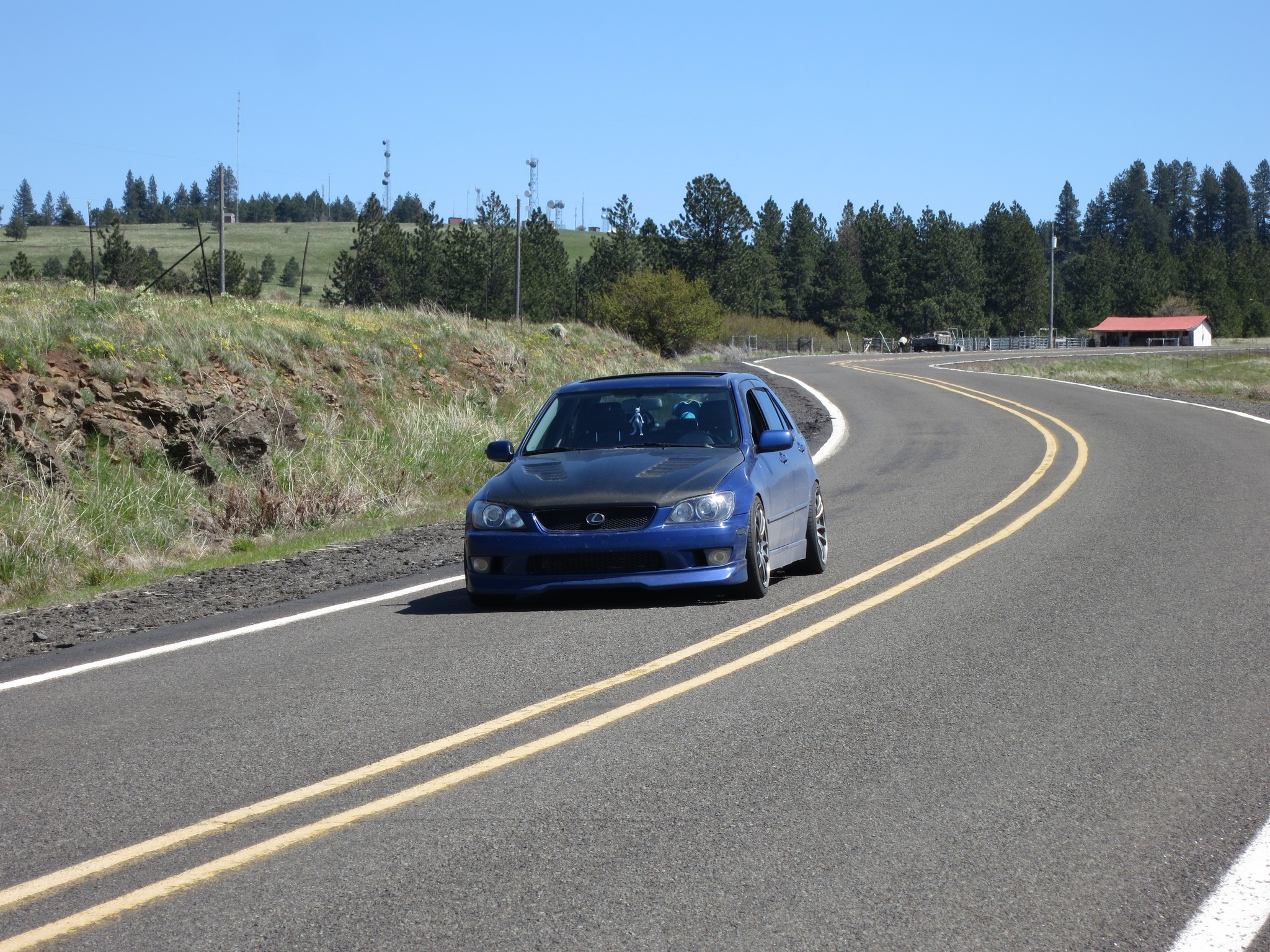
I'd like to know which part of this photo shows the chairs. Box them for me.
[700,400,735,444]
[583,403,627,448]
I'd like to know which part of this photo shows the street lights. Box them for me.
[1047,235,1057,349]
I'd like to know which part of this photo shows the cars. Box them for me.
[1044,336,1068,346]
[461,371,827,607]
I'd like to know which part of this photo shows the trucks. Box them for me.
[912,331,955,352]
